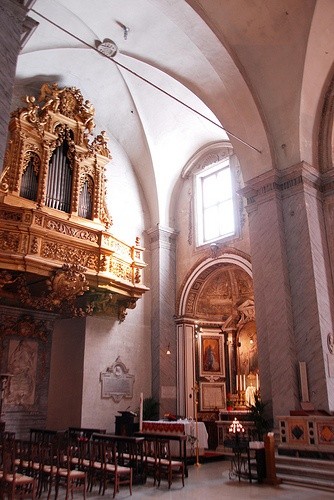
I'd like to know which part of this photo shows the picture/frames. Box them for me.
[199,333,225,377]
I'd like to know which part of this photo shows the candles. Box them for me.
[236,374,246,391]
[140,392,143,430]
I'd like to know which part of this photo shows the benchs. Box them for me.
[0,428,186,500]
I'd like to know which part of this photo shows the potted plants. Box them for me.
[243,389,272,451]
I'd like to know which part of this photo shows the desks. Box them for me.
[215,410,256,450]
[140,419,209,458]
